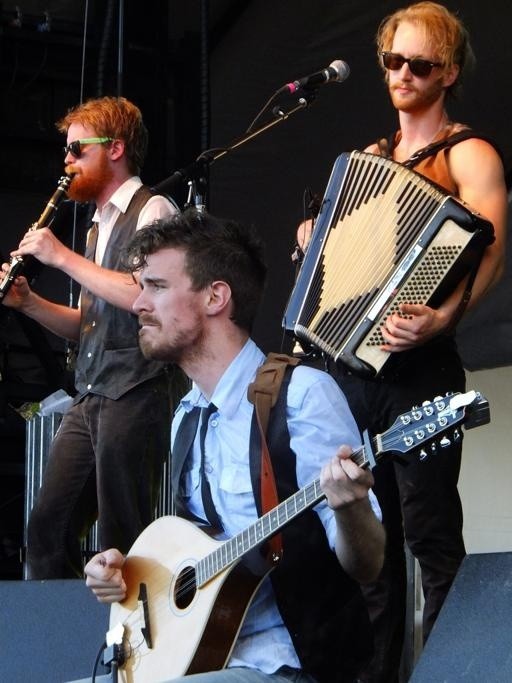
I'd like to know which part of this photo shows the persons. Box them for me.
[296,1,512,649]
[0,94,186,581]
[67,204,387,683]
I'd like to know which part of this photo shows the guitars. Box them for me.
[107,390,491,682]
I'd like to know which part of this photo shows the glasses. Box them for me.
[378,49,444,78]
[63,138,112,158]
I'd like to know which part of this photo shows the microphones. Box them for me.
[275,59,351,97]
